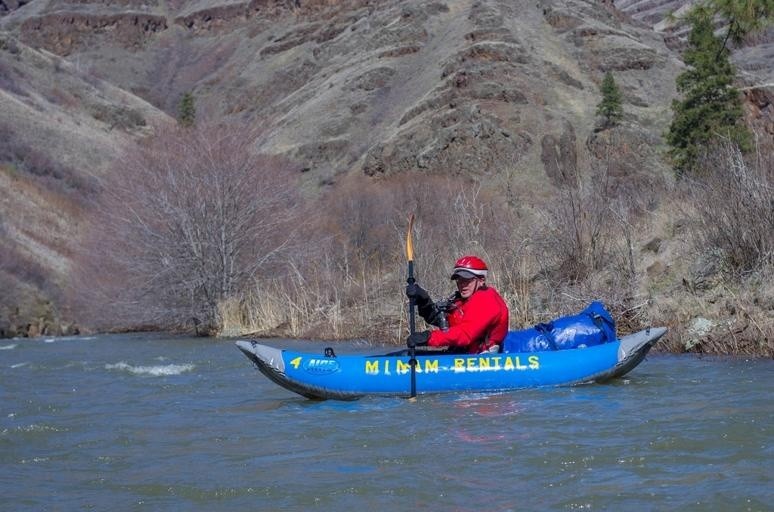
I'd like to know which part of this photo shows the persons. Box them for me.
[324,256,508,359]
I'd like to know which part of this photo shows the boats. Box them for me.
[234,326,669,402]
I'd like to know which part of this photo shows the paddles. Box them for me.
[407,214,415,404]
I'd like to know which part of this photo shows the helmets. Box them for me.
[454,255,489,279]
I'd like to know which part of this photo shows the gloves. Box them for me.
[407,330,430,347]
[406,284,434,317]
